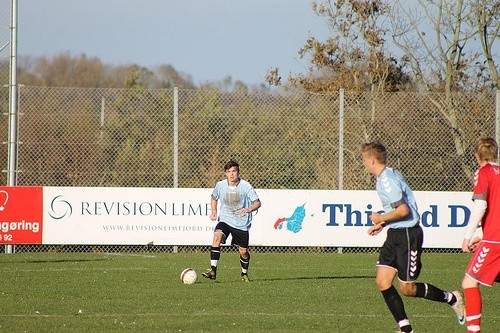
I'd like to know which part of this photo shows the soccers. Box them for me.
[179,268,197,284]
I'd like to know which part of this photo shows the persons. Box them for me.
[361,140,466,333]
[461,137,500,332]
[202,159,261,282]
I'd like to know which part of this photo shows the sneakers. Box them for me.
[202,270,216,280]
[451,291,466,326]
[242,275,251,283]
